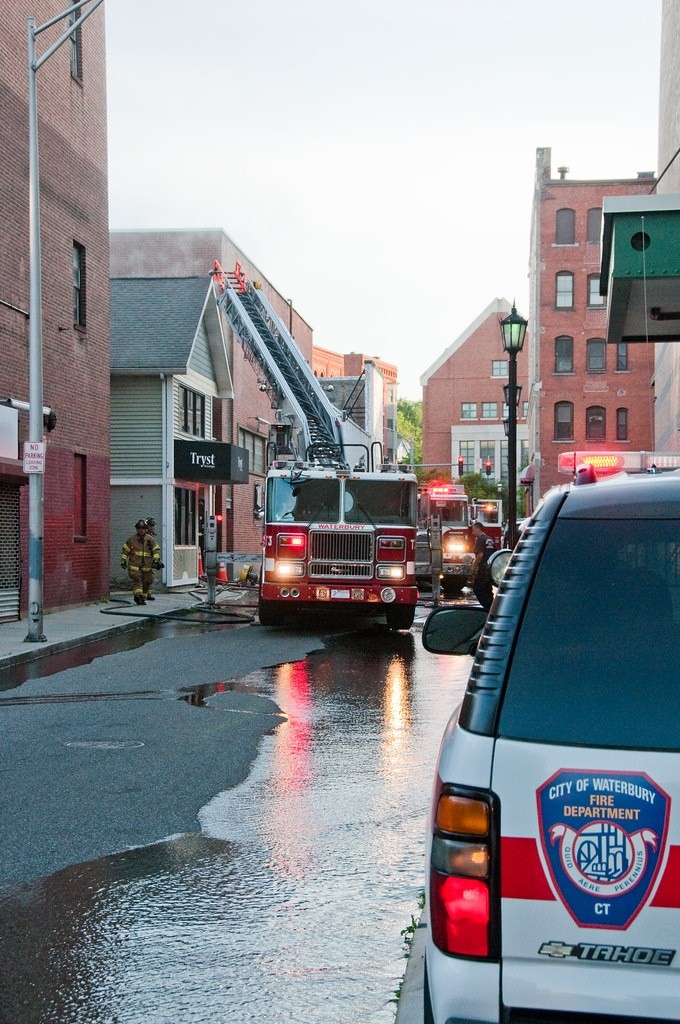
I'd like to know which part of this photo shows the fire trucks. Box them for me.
[414,477,502,599]
[209,259,419,630]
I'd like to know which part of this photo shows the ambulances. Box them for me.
[394,448,680,1024]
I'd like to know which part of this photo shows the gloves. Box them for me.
[121,562,128,569]
[151,557,165,570]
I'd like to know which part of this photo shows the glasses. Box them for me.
[148,524,154,526]
[138,527,147,529]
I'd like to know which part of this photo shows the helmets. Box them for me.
[145,517,157,524]
[134,519,148,529]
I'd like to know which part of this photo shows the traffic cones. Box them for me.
[198,546,203,577]
[218,562,228,581]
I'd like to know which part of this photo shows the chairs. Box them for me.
[621,567,672,625]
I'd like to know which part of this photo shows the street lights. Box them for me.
[497,298,528,551]
[501,417,509,437]
[501,382,522,406]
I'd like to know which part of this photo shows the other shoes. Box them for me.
[133,595,146,606]
[142,594,146,600]
[147,594,155,600]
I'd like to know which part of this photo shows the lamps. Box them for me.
[322,385,334,392]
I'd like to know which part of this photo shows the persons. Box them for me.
[120,517,165,607]
[504,521,523,553]
[471,521,498,613]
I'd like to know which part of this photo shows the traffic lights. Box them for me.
[485,460,491,476]
[458,456,463,476]
